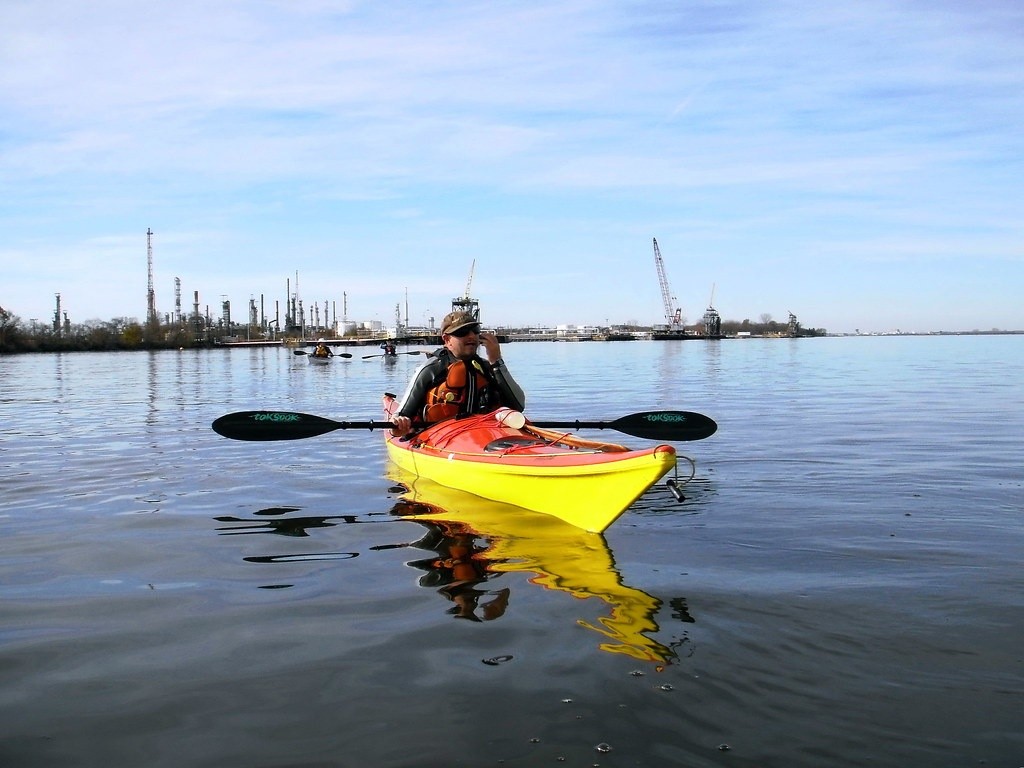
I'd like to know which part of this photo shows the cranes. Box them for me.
[701,282,723,335]
[451,257,481,329]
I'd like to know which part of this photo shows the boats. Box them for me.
[307,352,333,365]
[384,352,399,365]
[591,332,610,341]
[380,390,679,535]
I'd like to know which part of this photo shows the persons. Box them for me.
[388,311,526,437]
[311,338,333,358]
[380,338,400,356]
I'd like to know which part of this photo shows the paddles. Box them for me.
[361,351,420,359]
[210,410,718,442]
[293,350,353,359]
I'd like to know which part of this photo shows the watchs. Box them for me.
[491,358,504,368]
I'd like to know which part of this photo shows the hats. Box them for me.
[318,338,327,343]
[441,313,483,338]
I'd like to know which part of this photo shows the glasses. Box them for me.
[449,325,481,338]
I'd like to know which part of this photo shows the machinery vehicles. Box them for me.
[651,237,686,334]
